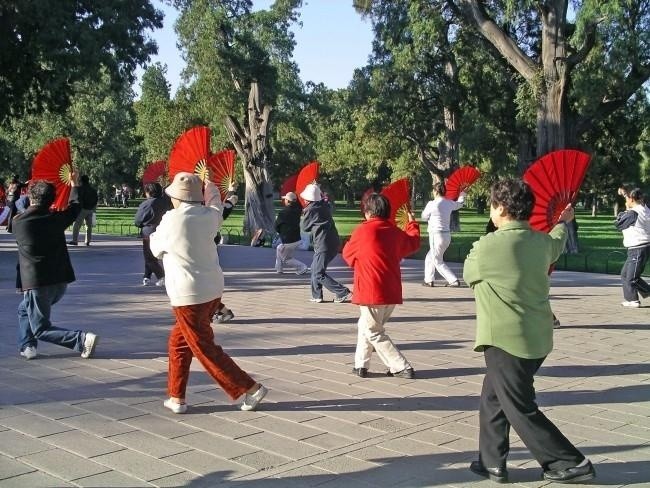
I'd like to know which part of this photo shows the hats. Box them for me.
[299,184,322,202]
[281,192,297,202]
[164,172,204,203]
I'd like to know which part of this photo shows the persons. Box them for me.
[420,181,465,288]
[300,184,353,303]
[212,182,239,323]
[149,173,266,414]
[342,193,420,378]
[0,174,169,295]
[274,192,308,275]
[464,177,595,482]
[548,264,561,329]
[12,171,99,358]
[614,188,650,308]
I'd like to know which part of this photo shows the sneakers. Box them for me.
[86,242,89,246]
[143,277,165,286]
[296,268,308,275]
[277,270,283,273]
[445,280,460,287]
[67,241,78,245]
[423,282,434,287]
[20,346,37,359]
[241,383,267,410]
[553,320,560,329]
[333,292,353,303]
[212,309,234,323]
[80,332,97,358]
[164,397,187,413]
[309,298,323,302]
[622,300,640,307]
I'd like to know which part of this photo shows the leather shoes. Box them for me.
[470,461,508,482]
[541,459,596,484]
[352,367,367,378]
[387,368,414,379]
